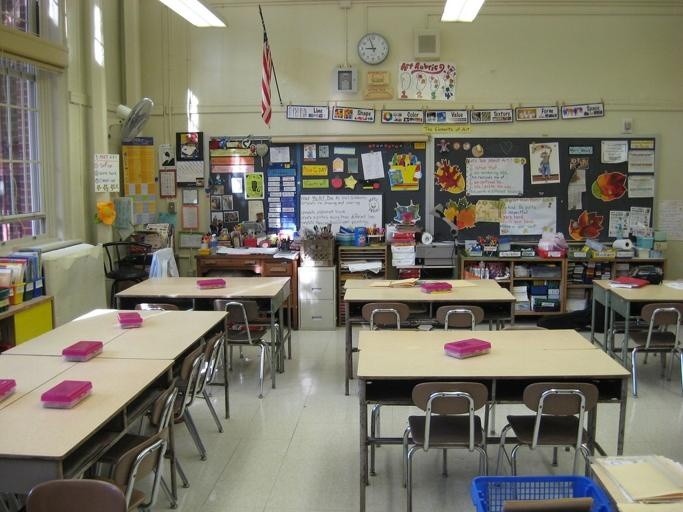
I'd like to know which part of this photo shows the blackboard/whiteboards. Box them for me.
[429,133,660,246]
[209,135,429,246]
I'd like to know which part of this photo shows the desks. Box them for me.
[339,276,517,400]
[0,252,296,512]
[585,277,681,399]
[355,329,631,512]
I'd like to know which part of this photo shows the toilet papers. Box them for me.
[612,239,632,250]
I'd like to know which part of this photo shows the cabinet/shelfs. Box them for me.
[297,267,337,331]
[337,247,682,329]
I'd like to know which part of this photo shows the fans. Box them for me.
[108,97,155,142]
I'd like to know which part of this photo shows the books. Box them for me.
[372,276,421,288]
[463,262,662,315]
[0,246,45,314]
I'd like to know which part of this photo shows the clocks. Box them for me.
[356,32,390,66]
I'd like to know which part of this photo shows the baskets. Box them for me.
[470,476,612,512]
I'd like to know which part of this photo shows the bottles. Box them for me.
[210,233,218,256]
